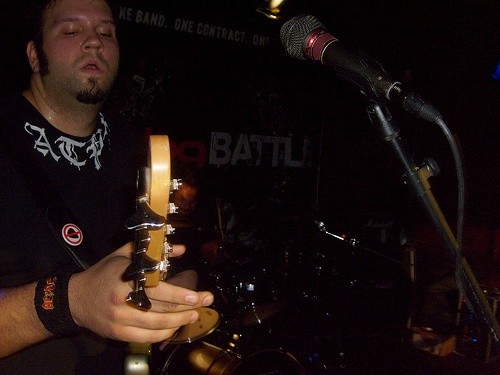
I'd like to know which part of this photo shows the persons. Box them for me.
[166,170,202,217]
[0,0,216,375]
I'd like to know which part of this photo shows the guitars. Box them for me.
[124,134,183,375]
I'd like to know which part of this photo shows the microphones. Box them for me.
[279,15,443,123]
